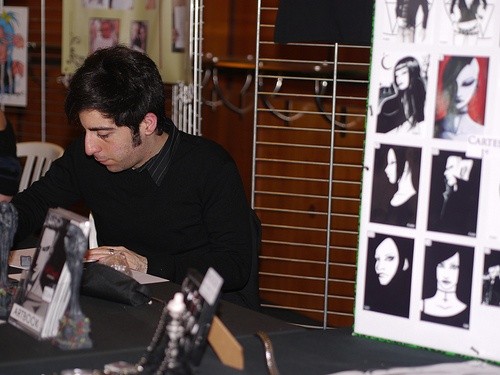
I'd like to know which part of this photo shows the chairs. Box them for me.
[16,142,65,194]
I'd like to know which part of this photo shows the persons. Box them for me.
[0,106,23,202]
[0,45,262,313]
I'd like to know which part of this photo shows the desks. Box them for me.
[0,274,499,375]
